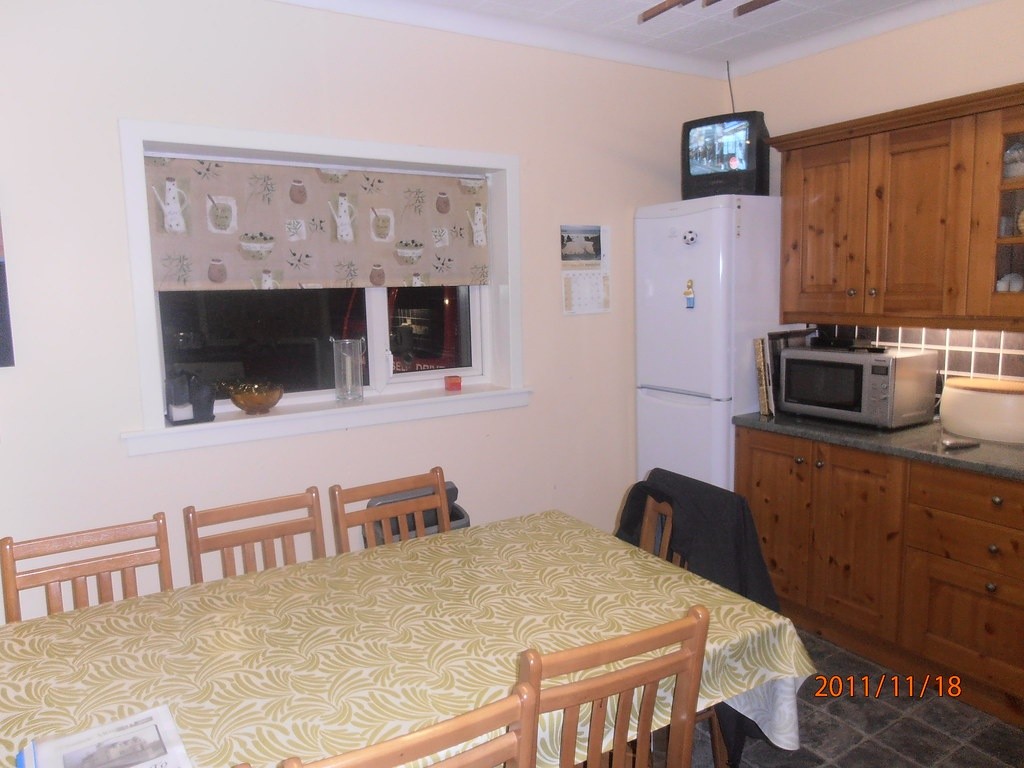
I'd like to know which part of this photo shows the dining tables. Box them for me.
[0,509,819,768]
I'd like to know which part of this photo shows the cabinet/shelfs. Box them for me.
[907,458,1024,730]
[976,81,1024,331]
[768,92,976,330]
[734,425,907,677]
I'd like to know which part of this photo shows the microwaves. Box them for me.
[779,348,936,427]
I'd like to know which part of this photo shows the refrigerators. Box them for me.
[636,194,779,491]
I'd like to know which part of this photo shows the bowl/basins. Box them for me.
[217,378,284,415]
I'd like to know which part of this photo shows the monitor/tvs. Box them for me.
[679,110,769,200]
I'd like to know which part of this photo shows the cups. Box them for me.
[333,340,363,402]
[999,216,1014,238]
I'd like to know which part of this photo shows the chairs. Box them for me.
[281,682,536,768]
[639,468,747,595]
[0,511,173,624]
[183,486,326,584]
[519,604,709,768]
[328,466,467,556]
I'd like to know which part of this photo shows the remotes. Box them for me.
[943,440,979,449]
